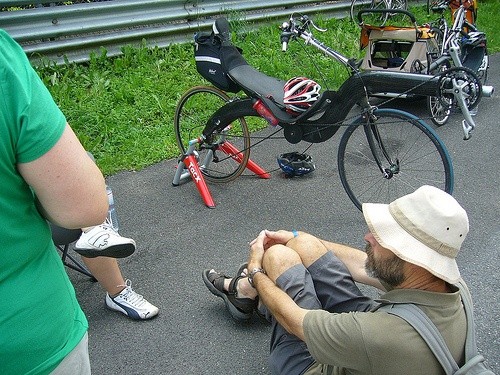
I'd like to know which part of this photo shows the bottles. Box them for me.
[102,184,119,231]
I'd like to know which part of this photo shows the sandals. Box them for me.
[237,262,274,319]
[202,268,259,322]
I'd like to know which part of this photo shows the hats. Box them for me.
[359,184,468,284]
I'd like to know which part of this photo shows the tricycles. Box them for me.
[360,0,488,127]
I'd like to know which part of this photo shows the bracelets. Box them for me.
[292,231,298,237]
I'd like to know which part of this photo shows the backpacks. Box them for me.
[376,282,495,375]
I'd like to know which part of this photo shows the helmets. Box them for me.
[283,77,321,115]
[277,151,314,174]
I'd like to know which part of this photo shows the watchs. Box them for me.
[248,268,267,289]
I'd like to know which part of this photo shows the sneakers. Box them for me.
[74,224,136,259]
[106,285,158,319]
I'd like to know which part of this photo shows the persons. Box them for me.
[44,152,159,321]
[0,29,109,374]
[202,185,474,375]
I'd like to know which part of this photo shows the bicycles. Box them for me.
[171,11,483,214]
[350,0,408,30]
